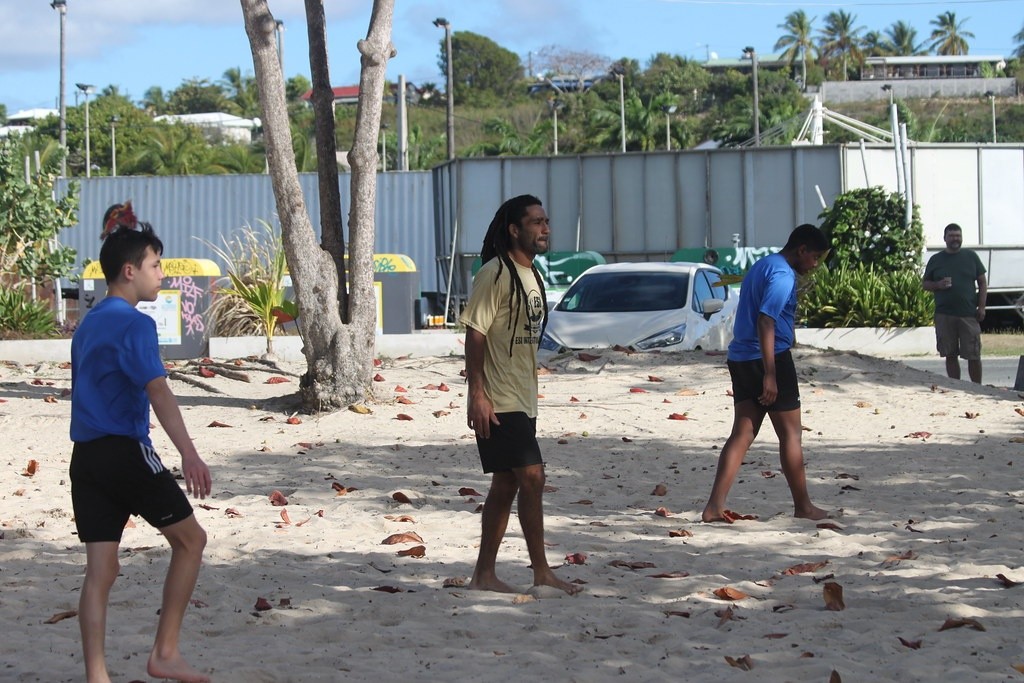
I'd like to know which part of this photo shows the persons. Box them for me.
[68,223,216,683]
[922,224,987,387]
[457,195,583,595]
[701,224,845,521]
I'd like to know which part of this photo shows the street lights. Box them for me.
[744,47,760,148]
[985,92,996,144]
[664,104,677,150]
[432,16,457,160]
[552,98,557,156]
[76,82,95,178]
[51,0,68,180]
[881,84,894,145]
[612,68,626,154]
[105,114,117,176]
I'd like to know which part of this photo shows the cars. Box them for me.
[538,263,743,355]
